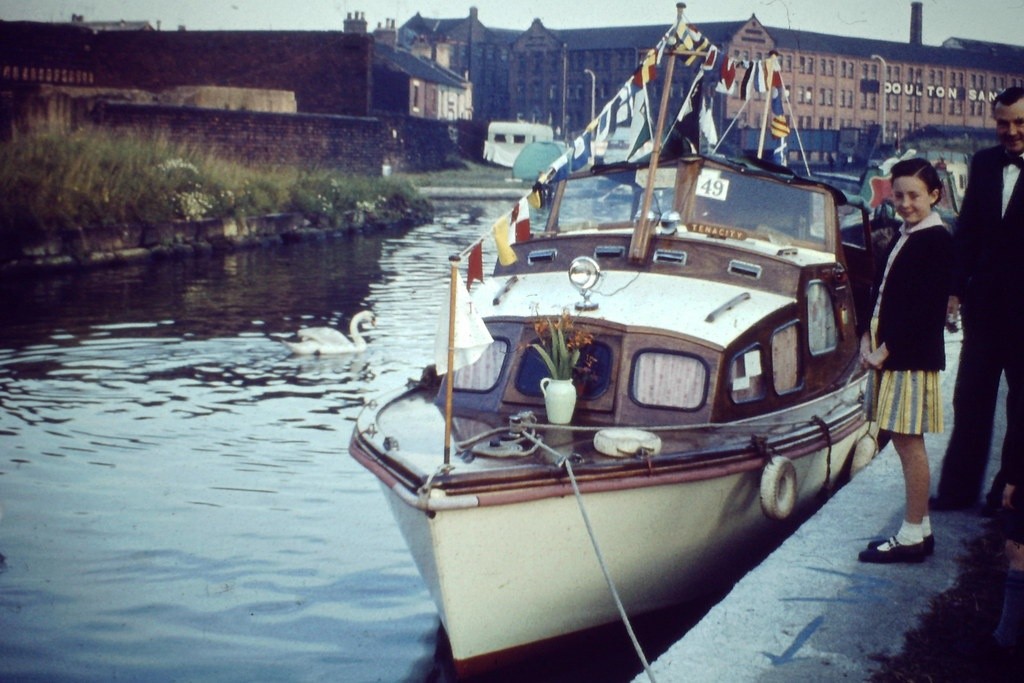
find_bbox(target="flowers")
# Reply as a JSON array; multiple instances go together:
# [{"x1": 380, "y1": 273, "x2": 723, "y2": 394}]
[{"x1": 519, "y1": 300, "x2": 598, "y2": 378}]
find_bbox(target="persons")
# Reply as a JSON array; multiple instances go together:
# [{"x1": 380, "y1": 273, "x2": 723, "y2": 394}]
[
  {"x1": 857, "y1": 157, "x2": 961, "y2": 562},
  {"x1": 927, "y1": 87, "x2": 1023, "y2": 683}
]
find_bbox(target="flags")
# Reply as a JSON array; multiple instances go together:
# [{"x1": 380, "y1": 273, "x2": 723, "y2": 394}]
[{"x1": 430, "y1": 12, "x2": 791, "y2": 379}]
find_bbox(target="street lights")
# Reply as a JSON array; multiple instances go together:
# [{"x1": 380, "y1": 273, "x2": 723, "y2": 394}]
[
  {"x1": 870, "y1": 53, "x2": 887, "y2": 147},
  {"x1": 583, "y1": 68, "x2": 595, "y2": 124}
]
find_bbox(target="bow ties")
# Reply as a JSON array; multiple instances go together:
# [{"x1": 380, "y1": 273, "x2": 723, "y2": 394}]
[{"x1": 998, "y1": 155, "x2": 1024, "y2": 170}]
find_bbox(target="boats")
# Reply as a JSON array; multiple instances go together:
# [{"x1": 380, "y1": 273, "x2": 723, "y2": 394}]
[
  {"x1": 348, "y1": 3, "x2": 962, "y2": 681},
  {"x1": 810, "y1": 147, "x2": 968, "y2": 223}
]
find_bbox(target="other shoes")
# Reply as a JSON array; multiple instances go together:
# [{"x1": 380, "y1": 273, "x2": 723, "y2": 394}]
[
  {"x1": 968, "y1": 636, "x2": 1020, "y2": 669},
  {"x1": 930, "y1": 490, "x2": 981, "y2": 511}
]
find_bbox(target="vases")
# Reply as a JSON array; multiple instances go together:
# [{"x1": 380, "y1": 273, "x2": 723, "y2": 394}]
[{"x1": 542, "y1": 380, "x2": 578, "y2": 423}]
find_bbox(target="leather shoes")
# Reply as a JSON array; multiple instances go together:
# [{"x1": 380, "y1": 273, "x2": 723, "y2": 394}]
[{"x1": 858, "y1": 534, "x2": 935, "y2": 562}]
